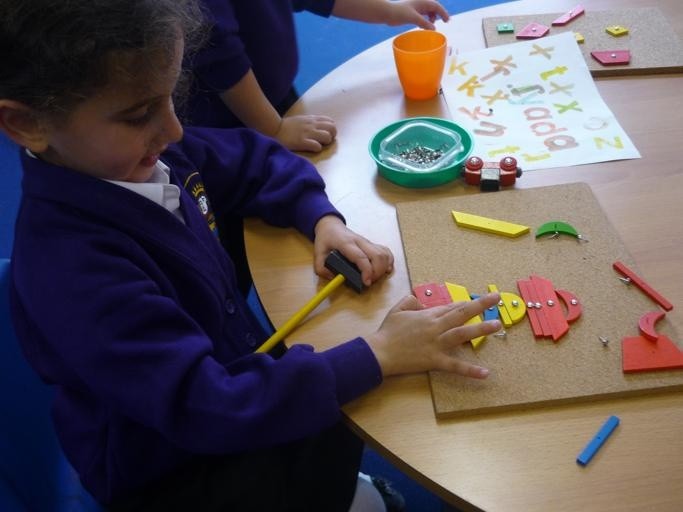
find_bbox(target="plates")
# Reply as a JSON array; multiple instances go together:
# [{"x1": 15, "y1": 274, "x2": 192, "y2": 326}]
[{"x1": 370, "y1": 117, "x2": 472, "y2": 188}]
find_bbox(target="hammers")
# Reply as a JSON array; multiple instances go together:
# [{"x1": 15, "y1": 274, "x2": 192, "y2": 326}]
[{"x1": 254, "y1": 249, "x2": 364, "y2": 355}]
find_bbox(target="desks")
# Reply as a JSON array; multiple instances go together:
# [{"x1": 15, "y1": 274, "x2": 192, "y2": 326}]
[{"x1": 241, "y1": 1, "x2": 683, "y2": 511}]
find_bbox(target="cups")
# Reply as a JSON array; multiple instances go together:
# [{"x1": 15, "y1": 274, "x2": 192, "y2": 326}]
[{"x1": 392, "y1": 28, "x2": 448, "y2": 101}]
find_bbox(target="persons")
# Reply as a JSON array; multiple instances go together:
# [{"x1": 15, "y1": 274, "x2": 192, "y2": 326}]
[
  {"x1": 173, "y1": 0, "x2": 451, "y2": 304},
  {"x1": 0, "y1": 0, "x2": 501, "y2": 510}
]
